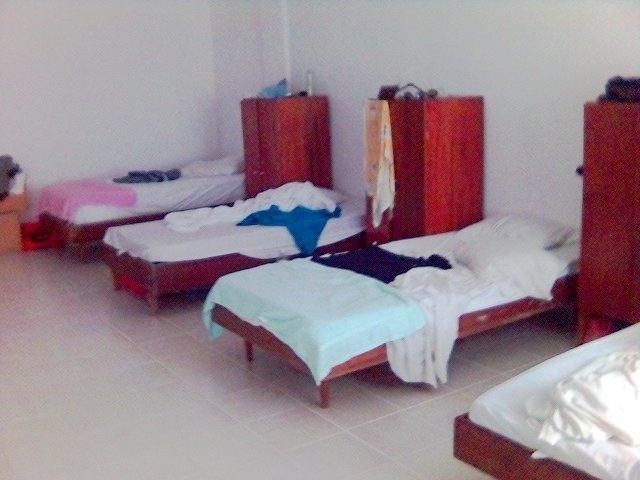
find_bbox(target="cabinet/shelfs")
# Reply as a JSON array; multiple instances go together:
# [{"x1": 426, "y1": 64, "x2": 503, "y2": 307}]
[
  {"x1": 364, "y1": 94, "x2": 486, "y2": 249},
  {"x1": 240, "y1": 94, "x2": 333, "y2": 200},
  {"x1": 576, "y1": 100, "x2": 640, "y2": 347}
]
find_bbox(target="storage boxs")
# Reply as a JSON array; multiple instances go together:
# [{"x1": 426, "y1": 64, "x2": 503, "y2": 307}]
[
  {"x1": 0, "y1": 212, "x2": 24, "y2": 255},
  {"x1": 0, "y1": 172, "x2": 28, "y2": 214},
  {"x1": 21, "y1": 222, "x2": 64, "y2": 250}
]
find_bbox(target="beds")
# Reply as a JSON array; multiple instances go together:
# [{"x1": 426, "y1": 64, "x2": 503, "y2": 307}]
[
  {"x1": 33, "y1": 172, "x2": 247, "y2": 263},
  {"x1": 201, "y1": 232, "x2": 581, "y2": 410},
  {"x1": 454, "y1": 320, "x2": 640, "y2": 480},
  {"x1": 96, "y1": 198, "x2": 366, "y2": 315}
]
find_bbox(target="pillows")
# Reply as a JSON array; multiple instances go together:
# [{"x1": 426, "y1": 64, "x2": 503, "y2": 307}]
[
  {"x1": 319, "y1": 188, "x2": 346, "y2": 204},
  {"x1": 181, "y1": 151, "x2": 245, "y2": 177},
  {"x1": 454, "y1": 211, "x2": 574, "y2": 250}
]
